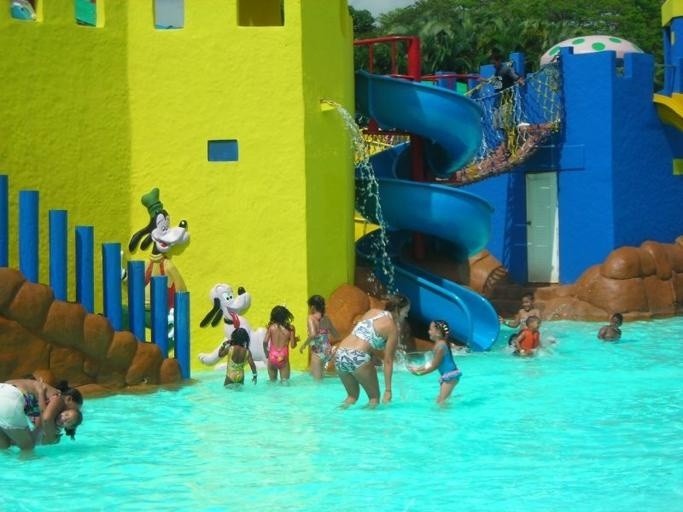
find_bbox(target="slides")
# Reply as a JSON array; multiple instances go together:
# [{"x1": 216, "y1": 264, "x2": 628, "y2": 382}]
[{"x1": 355, "y1": 69, "x2": 500, "y2": 352}]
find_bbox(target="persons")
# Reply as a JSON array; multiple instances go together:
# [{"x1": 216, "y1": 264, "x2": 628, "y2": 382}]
[
  {"x1": 409, "y1": 320, "x2": 462, "y2": 402},
  {"x1": 475, "y1": 47, "x2": 525, "y2": 163},
  {"x1": 335, "y1": 295, "x2": 410, "y2": 405},
  {"x1": 218, "y1": 328, "x2": 257, "y2": 385},
  {"x1": 499, "y1": 293, "x2": 541, "y2": 354},
  {"x1": 263, "y1": 305, "x2": 299, "y2": 381},
  {"x1": 598, "y1": 313, "x2": 623, "y2": 342},
  {"x1": 300, "y1": 295, "x2": 337, "y2": 381},
  {"x1": 0, "y1": 379, "x2": 82, "y2": 451}
]
